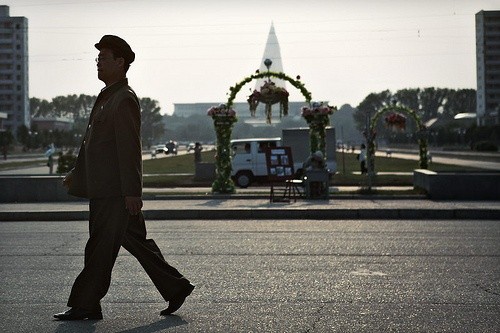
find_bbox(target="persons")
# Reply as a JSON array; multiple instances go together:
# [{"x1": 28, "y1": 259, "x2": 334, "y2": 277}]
[
  {"x1": 47, "y1": 151, "x2": 74, "y2": 175},
  {"x1": 358, "y1": 145, "x2": 369, "y2": 173},
  {"x1": 53, "y1": 35, "x2": 195, "y2": 320},
  {"x1": 193, "y1": 142, "x2": 202, "y2": 163},
  {"x1": 302, "y1": 150, "x2": 325, "y2": 198}
]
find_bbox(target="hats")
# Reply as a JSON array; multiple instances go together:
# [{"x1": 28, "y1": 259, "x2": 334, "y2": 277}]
[{"x1": 95, "y1": 35, "x2": 135, "y2": 63}]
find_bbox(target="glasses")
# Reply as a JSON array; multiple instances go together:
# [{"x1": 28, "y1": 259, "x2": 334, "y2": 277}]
[{"x1": 96, "y1": 57, "x2": 119, "y2": 62}]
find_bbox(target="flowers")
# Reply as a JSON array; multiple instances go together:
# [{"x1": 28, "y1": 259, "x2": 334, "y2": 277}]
[
  {"x1": 208, "y1": 104, "x2": 235, "y2": 116},
  {"x1": 302, "y1": 106, "x2": 330, "y2": 116},
  {"x1": 247, "y1": 81, "x2": 289, "y2": 124}
]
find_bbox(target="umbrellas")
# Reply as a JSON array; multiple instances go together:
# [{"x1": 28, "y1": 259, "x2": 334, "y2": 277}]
[{"x1": 44, "y1": 148, "x2": 55, "y2": 156}]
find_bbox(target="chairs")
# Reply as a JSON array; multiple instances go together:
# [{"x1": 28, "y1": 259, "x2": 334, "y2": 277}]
[{"x1": 284, "y1": 169, "x2": 305, "y2": 202}]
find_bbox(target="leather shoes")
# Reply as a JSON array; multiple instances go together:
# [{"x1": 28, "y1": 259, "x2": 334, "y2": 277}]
[
  {"x1": 54, "y1": 307, "x2": 103, "y2": 321},
  {"x1": 160, "y1": 284, "x2": 195, "y2": 315}
]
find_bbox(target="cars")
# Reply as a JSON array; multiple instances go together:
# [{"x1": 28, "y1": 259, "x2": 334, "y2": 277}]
[
  {"x1": 155, "y1": 144, "x2": 166, "y2": 153},
  {"x1": 164, "y1": 143, "x2": 177, "y2": 155},
  {"x1": 187, "y1": 143, "x2": 195, "y2": 152}
]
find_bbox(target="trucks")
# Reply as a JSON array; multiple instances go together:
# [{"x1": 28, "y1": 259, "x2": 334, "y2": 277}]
[{"x1": 228, "y1": 127, "x2": 338, "y2": 188}]
